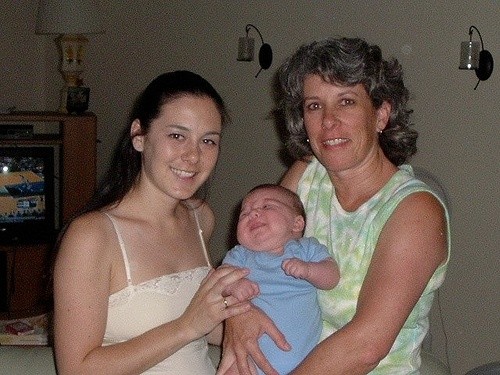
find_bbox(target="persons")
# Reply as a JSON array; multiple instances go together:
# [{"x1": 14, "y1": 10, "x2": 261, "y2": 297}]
[
  {"x1": 212, "y1": 37, "x2": 452, "y2": 375},
  {"x1": 53, "y1": 69, "x2": 251, "y2": 375},
  {"x1": 215, "y1": 183, "x2": 340, "y2": 375}
]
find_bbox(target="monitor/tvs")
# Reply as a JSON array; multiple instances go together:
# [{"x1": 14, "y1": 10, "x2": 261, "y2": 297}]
[{"x1": 0, "y1": 143, "x2": 62, "y2": 239}]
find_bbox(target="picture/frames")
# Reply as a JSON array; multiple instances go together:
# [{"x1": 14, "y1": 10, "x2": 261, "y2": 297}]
[{"x1": 66, "y1": 86, "x2": 90, "y2": 112}]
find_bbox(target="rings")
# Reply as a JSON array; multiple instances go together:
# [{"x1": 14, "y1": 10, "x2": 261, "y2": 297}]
[{"x1": 223, "y1": 298, "x2": 229, "y2": 309}]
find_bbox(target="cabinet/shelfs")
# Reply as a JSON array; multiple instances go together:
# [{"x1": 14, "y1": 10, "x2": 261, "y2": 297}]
[{"x1": 0, "y1": 112, "x2": 97, "y2": 317}]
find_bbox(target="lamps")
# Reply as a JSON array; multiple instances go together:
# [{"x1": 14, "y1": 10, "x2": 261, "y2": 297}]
[
  {"x1": 237, "y1": 24, "x2": 273, "y2": 79},
  {"x1": 459, "y1": 25, "x2": 494, "y2": 90},
  {"x1": 36, "y1": 0, "x2": 108, "y2": 115}
]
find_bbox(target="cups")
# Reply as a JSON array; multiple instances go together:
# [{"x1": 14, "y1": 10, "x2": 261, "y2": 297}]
[{"x1": 66, "y1": 85, "x2": 90, "y2": 115}]
